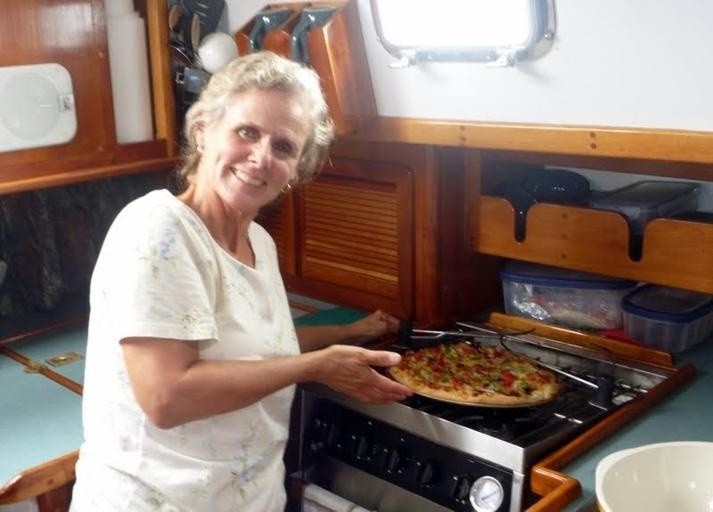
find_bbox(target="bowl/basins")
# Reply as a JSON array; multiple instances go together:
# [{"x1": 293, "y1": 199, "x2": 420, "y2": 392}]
[{"x1": 593, "y1": 440, "x2": 713, "y2": 512}]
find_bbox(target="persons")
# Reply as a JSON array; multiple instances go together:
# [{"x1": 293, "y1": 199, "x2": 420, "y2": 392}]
[{"x1": 63, "y1": 48, "x2": 415, "y2": 511}]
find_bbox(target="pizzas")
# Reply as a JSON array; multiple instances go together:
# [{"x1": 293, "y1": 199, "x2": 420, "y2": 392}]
[{"x1": 390, "y1": 342, "x2": 561, "y2": 406}]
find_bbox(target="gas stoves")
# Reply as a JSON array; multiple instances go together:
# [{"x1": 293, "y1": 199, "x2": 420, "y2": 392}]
[{"x1": 294, "y1": 315, "x2": 657, "y2": 512}]
